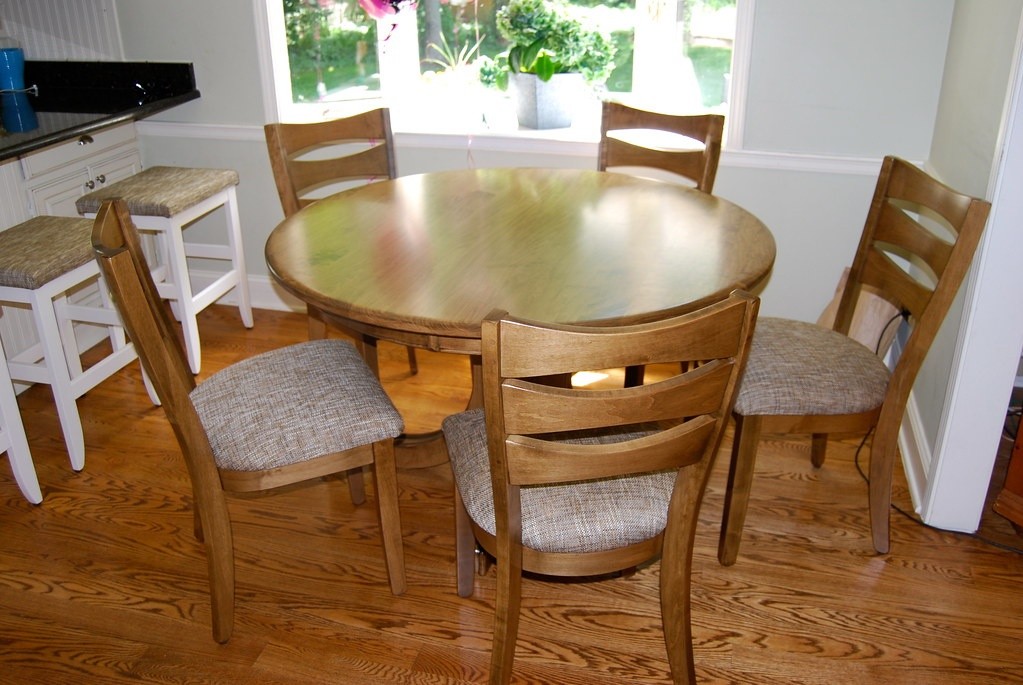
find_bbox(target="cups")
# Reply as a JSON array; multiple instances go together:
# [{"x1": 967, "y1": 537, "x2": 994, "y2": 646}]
[
  {"x1": 1, "y1": 49, "x2": 24, "y2": 93},
  {"x1": 3, "y1": 94, "x2": 38, "y2": 131}
]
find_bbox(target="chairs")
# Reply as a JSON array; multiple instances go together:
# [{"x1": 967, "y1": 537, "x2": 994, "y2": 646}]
[
  {"x1": 265, "y1": 98, "x2": 991, "y2": 567},
  {"x1": 88, "y1": 195, "x2": 407, "y2": 643},
  {"x1": 441, "y1": 289, "x2": 761, "y2": 685}
]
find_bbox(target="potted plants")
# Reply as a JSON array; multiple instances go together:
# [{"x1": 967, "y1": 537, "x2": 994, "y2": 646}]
[{"x1": 421, "y1": 1, "x2": 617, "y2": 131}]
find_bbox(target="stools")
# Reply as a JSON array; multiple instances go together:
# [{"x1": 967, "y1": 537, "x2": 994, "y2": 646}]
[
  {"x1": 75, "y1": 165, "x2": 253, "y2": 375},
  {"x1": 0, "y1": 215, "x2": 161, "y2": 505}
]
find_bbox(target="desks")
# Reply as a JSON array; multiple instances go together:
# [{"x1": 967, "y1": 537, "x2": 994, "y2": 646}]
[{"x1": 265, "y1": 166, "x2": 776, "y2": 577}]
